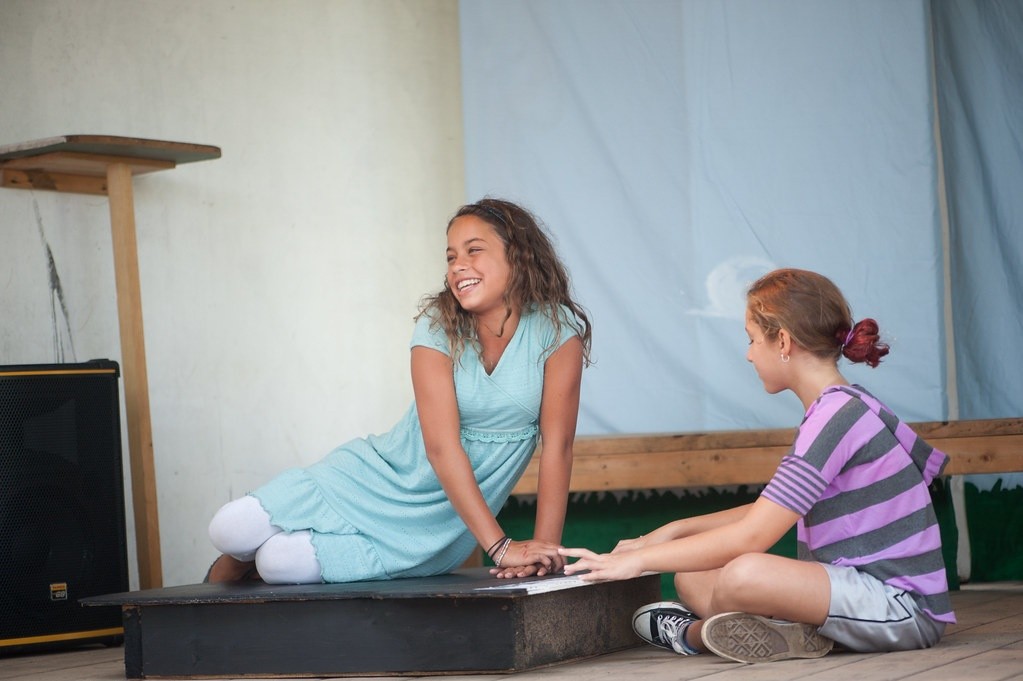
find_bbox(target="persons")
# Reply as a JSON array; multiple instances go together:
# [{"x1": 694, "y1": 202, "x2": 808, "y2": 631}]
[
  {"x1": 200, "y1": 199, "x2": 597, "y2": 586},
  {"x1": 558, "y1": 267, "x2": 957, "y2": 665}
]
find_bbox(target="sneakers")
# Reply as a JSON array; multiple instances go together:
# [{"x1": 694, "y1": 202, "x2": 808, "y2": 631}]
[
  {"x1": 701, "y1": 611, "x2": 834, "y2": 663},
  {"x1": 632, "y1": 601, "x2": 699, "y2": 655}
]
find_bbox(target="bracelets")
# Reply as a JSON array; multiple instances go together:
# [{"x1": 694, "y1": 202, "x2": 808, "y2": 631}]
[{"x1": 487, "y1": 535, "x2": 513, "y2": 567}]
